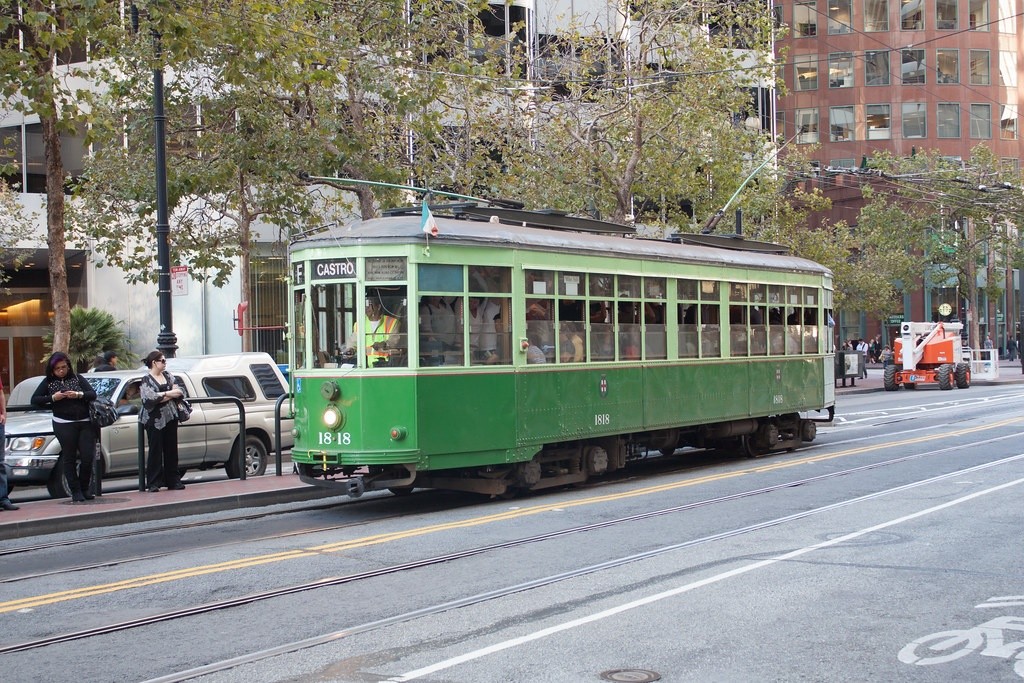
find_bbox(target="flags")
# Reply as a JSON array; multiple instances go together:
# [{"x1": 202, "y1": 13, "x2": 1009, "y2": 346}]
[{"x1": 420, "y1": 200, "x2": 439, "y2": 237}]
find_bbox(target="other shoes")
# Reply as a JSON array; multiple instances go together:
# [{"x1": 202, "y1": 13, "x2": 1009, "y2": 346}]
[
  {"x1": 83, "y1": 493, "x2": 95, "y2": 499},
  {"x1": 150, "y1": 486, "x2": 159, "y2": 492},
  {"x1": 72, "y1": 494, "x2": 85, "y2": 501},
  {"x1": 865, "y1": 375, "x2": 867, "y2": 378},
  {"x1": 0, "y1": 499, "x2": 19, "y2": 511},
  {"x1": 858, "y1": 378, "x2": 863, "y2": 379},
  {"x1": 168, "y1": 485, "x2": 185, "y2": 489}
]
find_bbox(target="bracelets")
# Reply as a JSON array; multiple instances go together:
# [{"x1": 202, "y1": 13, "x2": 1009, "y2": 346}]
[
  {"x1": 165, "y1": 390, "x2": 166, "y2": 396},
  {"x1": 76, "y1": 391, "x2": 78, "y2": 398},
  {"x1": 52, "y1": 395, "x2": 55, "y2": 402}
]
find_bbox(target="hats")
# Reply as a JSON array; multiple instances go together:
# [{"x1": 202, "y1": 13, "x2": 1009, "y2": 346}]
[
  {"x1": 104, "y1": 351, "x2": 118, "y2": 359},
  {"x1": 98, "y1": 352, "x2": 105, "y2": 357}
]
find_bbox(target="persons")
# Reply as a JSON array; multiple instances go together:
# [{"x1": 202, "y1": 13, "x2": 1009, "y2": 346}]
[
  {"x1": 138, "y1": 351, "x2": 185, "y2": 492},
  {"x1": 87, "y1": 351, "x2": 119, "y2": 373},
  {"x1": 879, "y1": 345, "x2": 894, "y2": 369},
  {"x1": 30, "y1": 352, "x2": 97, "y2": 502},
  {"x1": 347, "y1": 288, "x2": 401, "y2": 368},
  {"x1": 0, "y1": 376, "x2": 20, "y2": 511},
  {"x1": 984, "y1": 335, "x2": 993, "y2": 360},
  {"x1": 841, "y1": 337, "x2": 868, "y2": 379},
  {"x1": 119, "y1": 383, "x2": 141, "y2": 412},
  {"x1": 868, "y1": 336, "x2": 882, "y2": 364},
  {"x1": 1006, "y1": 336, "x2": 1018, "y2": 361},
  {"x1": 419, "y1": 265, "x2": 835, "y2": 363}
]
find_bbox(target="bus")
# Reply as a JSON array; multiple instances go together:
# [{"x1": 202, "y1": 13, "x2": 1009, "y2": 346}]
[{"x1": 287, "y1": 202, "x2": 835, "y2": 497}]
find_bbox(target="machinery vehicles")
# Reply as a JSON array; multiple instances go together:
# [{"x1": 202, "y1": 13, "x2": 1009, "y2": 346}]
[{"x1": 883, "y1": 321, "x2": 971, "y2": 389}]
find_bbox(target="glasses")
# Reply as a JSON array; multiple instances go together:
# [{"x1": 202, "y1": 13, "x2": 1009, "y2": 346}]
[
  {"x1": 156, "y1": 359, "x2": 165, "y2": 364},
  {"x1": 53, "y1": 365, "x2": 68, "y2": 371}
]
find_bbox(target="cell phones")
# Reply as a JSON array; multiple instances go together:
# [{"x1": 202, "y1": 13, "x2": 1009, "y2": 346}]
[{"x1": 60, "y1": 389, "x2": 71, "y2": 394}]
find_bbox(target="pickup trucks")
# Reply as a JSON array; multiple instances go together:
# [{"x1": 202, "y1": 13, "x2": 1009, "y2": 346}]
[{"x1": 2, "y1": 353, "x2": 295, "y2": 498}]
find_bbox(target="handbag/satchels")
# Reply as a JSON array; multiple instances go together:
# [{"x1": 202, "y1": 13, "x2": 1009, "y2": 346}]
[
  {"x1": 163, "y1": 372, "x2": 192, "y2": 423},
  {"x1": 74, "y1": 372, "x2": 119, "y2": 426}
]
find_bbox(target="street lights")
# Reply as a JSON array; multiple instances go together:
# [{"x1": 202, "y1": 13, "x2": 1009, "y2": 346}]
[{"x1": 144, "y1": 0, "x2": 179, "y2": 358}]
